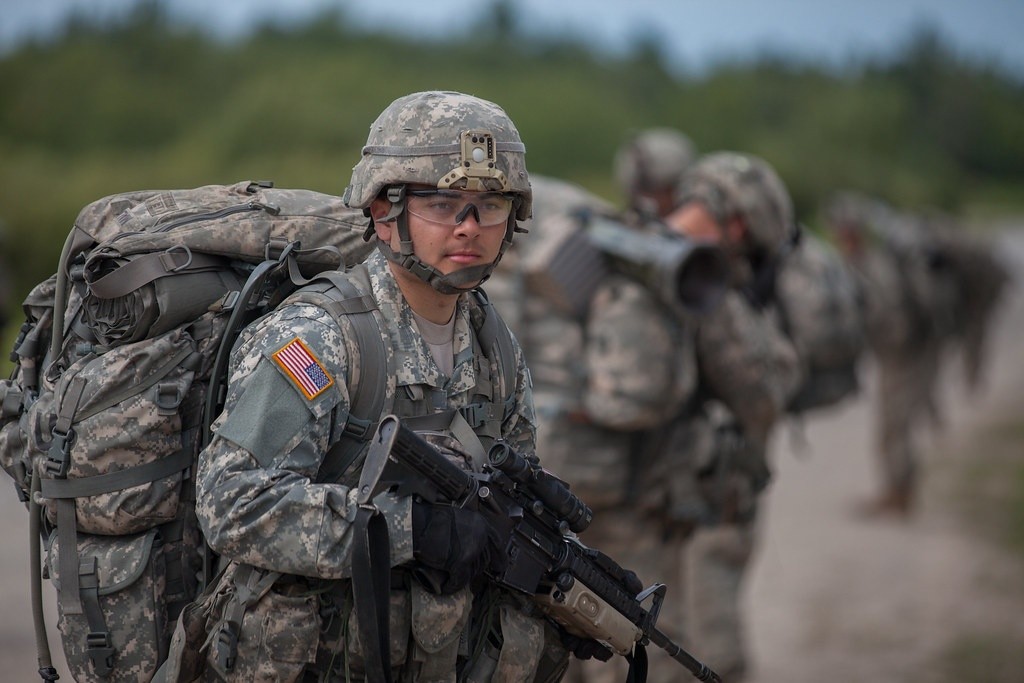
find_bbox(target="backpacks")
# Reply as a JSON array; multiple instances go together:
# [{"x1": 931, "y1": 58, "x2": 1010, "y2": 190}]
[
  {"x1": 0, "y1": 179, "x2": 518, "y2": 683},
  {"x1": 484, "y1": 173, "x2": 698, "y2": 507},
  {"x1": 774, "y1": 224, "x2": 861, "y2": 410}
]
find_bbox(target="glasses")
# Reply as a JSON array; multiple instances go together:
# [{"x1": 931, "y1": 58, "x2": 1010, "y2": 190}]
[{"x1": 377, "y1": 189, "x2": 516, "y2": 227}]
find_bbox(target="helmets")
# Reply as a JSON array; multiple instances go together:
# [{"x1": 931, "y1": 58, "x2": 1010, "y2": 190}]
[
  {"x1": 671, "y1": 148, "x2": 796, "y2": 250},
  {"x1": 615, "y1": 126, "x2": 697, "y2": 199},
  {"x1": 343, "y1": 90, "x2": 533, "y2": 221}
]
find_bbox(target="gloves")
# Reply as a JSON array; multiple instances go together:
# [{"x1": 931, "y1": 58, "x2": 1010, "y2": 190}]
[
  {"x1": 558, "y1": 569, "x2": 643, "y2": 663},
  {"x1": 418, "y1": 502, "x2": 515, "y2": 596}
]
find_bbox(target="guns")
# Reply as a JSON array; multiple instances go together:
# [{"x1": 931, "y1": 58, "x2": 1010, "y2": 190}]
[{"x1": 354, "y1": 415, "x2": 727, "y2": 683}]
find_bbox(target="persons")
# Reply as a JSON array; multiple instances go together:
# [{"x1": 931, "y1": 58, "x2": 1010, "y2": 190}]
[
  {"x1": 575, "y1": 127, "x2": 943, "y2": 683},
  {"x1": 193, "y1": 91, "x2": 642, "y2": 683}
]
